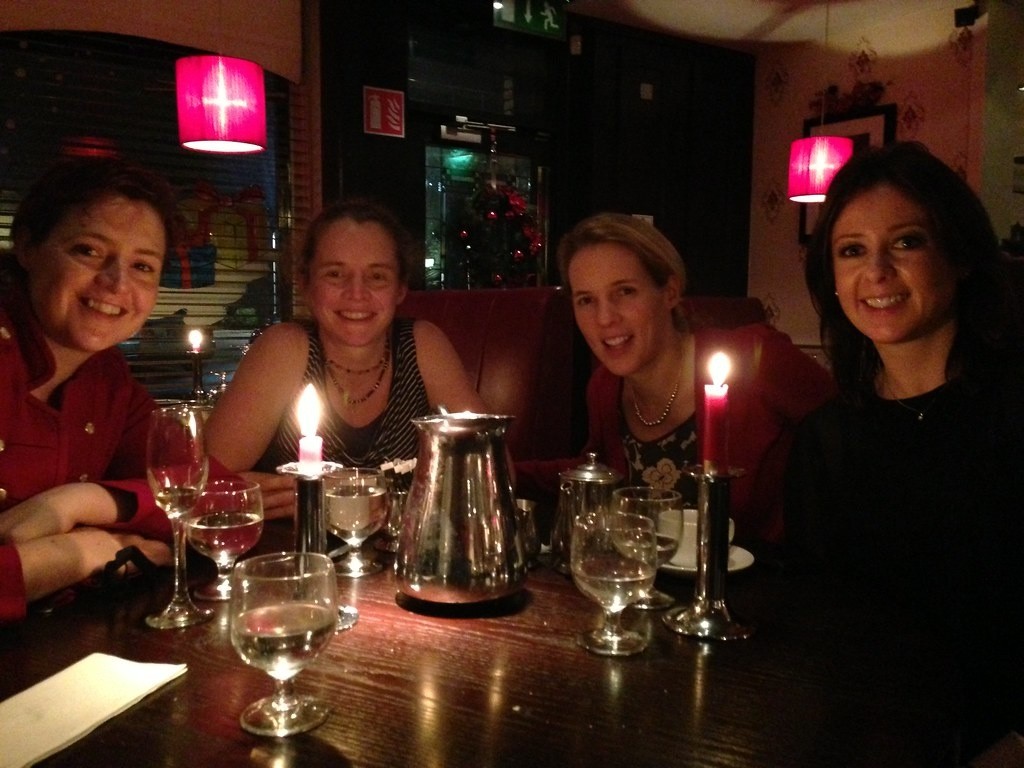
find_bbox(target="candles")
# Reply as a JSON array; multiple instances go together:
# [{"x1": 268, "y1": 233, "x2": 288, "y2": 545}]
[
  {"x1": 704, "y1": 384, "x2": 729, "y2": 473},
  {"x1": 299, "y1": 436, "x2": 322, "y2": 472}
]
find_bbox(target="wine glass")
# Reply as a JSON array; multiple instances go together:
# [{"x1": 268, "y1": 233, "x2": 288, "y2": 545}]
[
  {"x1": 322, "y1": 468, "x2": 389, "y2": 579},
  {"x1": 611, "y1": 486, "x2": 684, "y2": 611},
  {"x1": 186, "y1": 480, "x2": 265, "y2": 601},
  {"x1": 229, "y1": 550, "x2": 340, "y2": 735},
  {"x1": 571, "y1": 512, "x2": 658, "y2": 657},
  {"x1": 145, "y1": 407, "x2": 215, "y2": 630}
]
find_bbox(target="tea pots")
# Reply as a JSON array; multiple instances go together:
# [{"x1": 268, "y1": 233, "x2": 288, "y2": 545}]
[{"x1": 550, "y1": 451, "x2": 625, "y2": 574}]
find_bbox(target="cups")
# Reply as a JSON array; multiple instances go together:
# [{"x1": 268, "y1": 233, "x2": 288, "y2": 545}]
[
  {"x1": 516, "y1": 497, "x2": 540, "y2": 568},
  {"x1": 658, "y1": 508, "x2": 734, "y2": 569}
]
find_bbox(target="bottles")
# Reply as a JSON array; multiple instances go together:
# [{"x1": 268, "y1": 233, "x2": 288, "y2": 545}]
[{"x1": 394, "y1": 411, "x2": 526, "y2": 607}]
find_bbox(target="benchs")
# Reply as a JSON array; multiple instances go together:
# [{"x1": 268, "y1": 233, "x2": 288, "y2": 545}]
[{"x1": 391, "y1": 288, "x2": 760, "y2": 526}]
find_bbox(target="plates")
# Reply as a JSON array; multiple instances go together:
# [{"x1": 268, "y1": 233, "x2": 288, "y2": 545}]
[{"x1": 661, "y1": 545, "x2": 755, "y2": 573}]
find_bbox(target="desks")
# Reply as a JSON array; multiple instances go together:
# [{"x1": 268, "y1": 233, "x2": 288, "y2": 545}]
[{"x1": 0, "y1": 520, "x2": 848, "y2": 768}]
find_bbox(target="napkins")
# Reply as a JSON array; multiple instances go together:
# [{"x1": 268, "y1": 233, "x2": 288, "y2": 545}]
[{"x1": 0, "y1": 652, "x2": 189, "y2": 768}]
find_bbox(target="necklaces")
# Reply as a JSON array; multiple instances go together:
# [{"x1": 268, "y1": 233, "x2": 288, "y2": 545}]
[
  {"x1": 887, "y1": 386, "x2": 926, "y2": 420},
  {"x1": 325, "y1": 329, "x2": 391, "y2": 404},
  {"x1": 631, "y1": 332, "x2": 685, "y2": 426}
]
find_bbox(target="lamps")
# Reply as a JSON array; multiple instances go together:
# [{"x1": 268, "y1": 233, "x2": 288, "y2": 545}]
[
  {"x1": 786, "y1": 0, "x2": 852, "y2": 203},
  {"x1": 175, "y1": 0, "x2": 267, "y2": 154}
]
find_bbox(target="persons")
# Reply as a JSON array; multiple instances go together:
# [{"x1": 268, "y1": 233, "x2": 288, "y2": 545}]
[
  {"x1": 782, "y1": 141, "x2": 1024, "y2": 768},
  {"x1": 202, "y1": 197, "x2": 486, "y2": 516},
  {"x1": 514, "y1": 212, "x2": 835, "y2": 542},
  {"x1": 0, "y1": 156, "x2": 244, "y2": 620}
]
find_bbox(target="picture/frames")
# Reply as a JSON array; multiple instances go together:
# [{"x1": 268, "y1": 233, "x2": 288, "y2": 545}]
[{"x1": 799, "y1": 103, "x2": 899, "y2": 242}]
